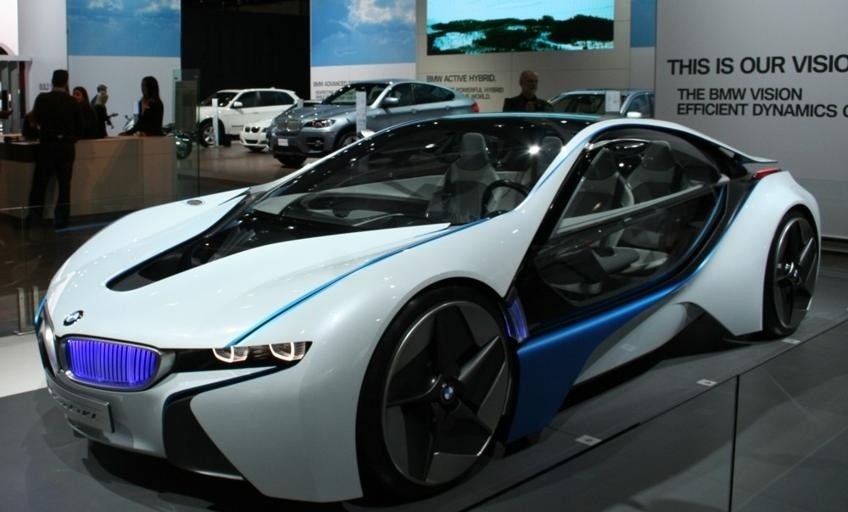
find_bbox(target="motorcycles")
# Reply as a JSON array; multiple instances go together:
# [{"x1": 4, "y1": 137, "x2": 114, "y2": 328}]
[{"x1": 119, "y1": 114, "x2": 193, "y2": 159}]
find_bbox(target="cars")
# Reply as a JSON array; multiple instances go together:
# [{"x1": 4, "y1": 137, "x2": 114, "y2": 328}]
[
  {"x1": 239, "y1": 100, "x2": 357, "y2": 152},
  {"x1": 265, "y1": 78, "x2": 481, "y2": 168},
  {"x1": 547, "y1": 87, "x2": 655, "y2": 117}
]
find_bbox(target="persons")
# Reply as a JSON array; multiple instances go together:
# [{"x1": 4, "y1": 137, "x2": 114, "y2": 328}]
[
  {"x1": 94, "y1": 95, "x2": 118, "y2": 130},
  {"x1": 92, "y1": 84, "x2": 119, "y2": 139},
  {"x1": 9, "y1": 68, "x2": 78, "y2": 232},
  {"x1": 0, "y1": 110, "x2": 13, "y2": 120},
  {"x1": 72, "y1": 85, "x2": 108, "y2": 140},
  {"x1": 500, "y1": 69, "x2": 556, "y2": 113},
  {"x1": 119, "y1": 76, "x2": 167, "y2": 136}
]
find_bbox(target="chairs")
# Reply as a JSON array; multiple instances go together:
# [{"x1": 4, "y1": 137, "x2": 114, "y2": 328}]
[
  {"x1": 426, "y1": 129, "x2": 501, "y2": 222},
  {"x1": 515, "y1": 135, "x2": 687, "y2": 278}
]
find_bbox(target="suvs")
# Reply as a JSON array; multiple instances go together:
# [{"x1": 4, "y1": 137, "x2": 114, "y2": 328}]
[{"x1": 195, "y1": 88, "x2": 301, "y2": 149}]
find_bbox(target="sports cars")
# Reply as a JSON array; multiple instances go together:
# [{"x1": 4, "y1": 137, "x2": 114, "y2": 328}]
[{"x1": 29, "y1": 112, "x2": 824, "y2": 508}]
